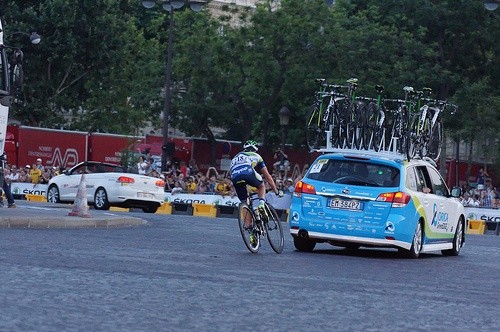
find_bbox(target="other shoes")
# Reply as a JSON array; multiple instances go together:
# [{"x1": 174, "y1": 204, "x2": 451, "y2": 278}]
[{"x1": 9, "y1": 203, "x2": 15, "y2": 207}]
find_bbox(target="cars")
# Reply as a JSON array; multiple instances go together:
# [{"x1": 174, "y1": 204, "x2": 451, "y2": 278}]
[{"x1": 287, "y1": 152, "x2": 466, "y2": 256}]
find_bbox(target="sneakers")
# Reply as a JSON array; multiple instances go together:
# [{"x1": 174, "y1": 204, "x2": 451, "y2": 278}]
[
  {"x1": 249, "y1": 235, "x2": 261, "y2": 247},
  {"x1": 258, "y1": 204, "x2": 269, "y2": 223}
]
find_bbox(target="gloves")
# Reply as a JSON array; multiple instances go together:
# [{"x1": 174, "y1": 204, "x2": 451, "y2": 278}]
[{"x1": 276, "y1": 190, "x2": 283, "y2": 198}]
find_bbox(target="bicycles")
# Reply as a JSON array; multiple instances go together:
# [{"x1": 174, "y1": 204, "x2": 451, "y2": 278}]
[
  {"x1": 236, "y1": 188, "x2": 285, "y2": 254},
  {"x1": 304, "y1": 78, "x2": 456, "y2": 162}
]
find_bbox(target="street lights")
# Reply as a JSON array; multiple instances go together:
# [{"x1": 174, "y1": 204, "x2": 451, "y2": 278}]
[{"x1": 142, "y1": 0, "x2": 207, "y2": 164}]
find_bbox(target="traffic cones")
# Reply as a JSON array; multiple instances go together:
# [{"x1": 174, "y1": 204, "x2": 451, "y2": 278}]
[{"x1": 69, "y1": 173, "x2": 93, "y2": 218}]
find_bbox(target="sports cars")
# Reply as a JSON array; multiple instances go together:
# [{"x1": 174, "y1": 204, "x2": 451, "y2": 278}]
[{"x1": 47, "y1": 162, "x2": 166, "y2": 214}]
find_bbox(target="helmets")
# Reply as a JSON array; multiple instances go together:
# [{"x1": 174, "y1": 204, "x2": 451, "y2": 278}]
[{"x1": 243, "y1": 140, "x2": 258, "y2": 151}]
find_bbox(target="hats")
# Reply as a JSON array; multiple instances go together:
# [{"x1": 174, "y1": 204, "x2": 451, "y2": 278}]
[
  {"x1": 26, "y1": 165, "x2": 30, "y2": 168},
  {"x1": 37, "y1": 158, "x2": 42, "y2": 162},
  {"x1": 288, "y1": 179, "x2": 292, "y2": 182}
]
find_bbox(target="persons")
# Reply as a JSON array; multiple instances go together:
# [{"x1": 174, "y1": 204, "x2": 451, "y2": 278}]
[
  {"x1": 0, "y1": 149, "x2": 304, "y2": 208},
  {"x1": 229, "y1": 140, "x2": 284, "y2": 247},
  {"x1": 356, "y1": 164, "x2": 500, "y2": 209}
]
